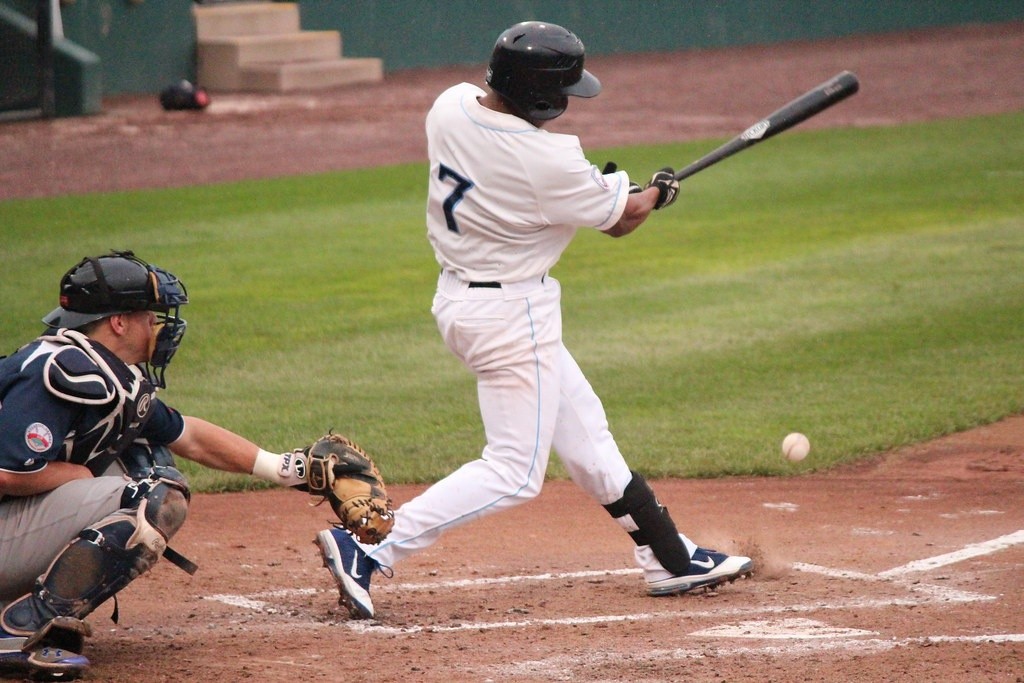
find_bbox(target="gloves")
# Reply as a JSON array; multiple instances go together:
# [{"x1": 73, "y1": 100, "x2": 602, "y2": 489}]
[
  {"x1": 643, "y1": 166, "x2": 681, "y2": 209},
  {"x1": 601, "y1": 161, "x2": 642, "y2": 195}
]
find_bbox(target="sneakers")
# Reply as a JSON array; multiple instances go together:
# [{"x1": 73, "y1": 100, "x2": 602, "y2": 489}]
[
  {"x1": 634, "y1": 531, "x2": 754, "y2": 597},
  {"x1": 312, "y1": 525, "x2": 393, "y2": 621},
  {"x1": 0, "y1": 629, "x2": 94, "y2": 678}
]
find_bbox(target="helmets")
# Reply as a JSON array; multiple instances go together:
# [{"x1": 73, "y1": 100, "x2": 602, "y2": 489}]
[
  {"x1": 41, "y1": 250, "x2": 163, "y2": 330},
  {"x1": 158, "y1": 78, "x2": 212, "y2": 110},
  {"x1": 485, "y1": 19, "x2": 603, "y2": 121}
]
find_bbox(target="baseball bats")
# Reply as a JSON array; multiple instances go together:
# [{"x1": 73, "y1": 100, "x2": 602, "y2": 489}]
[{"x1": 644, "y1": 68, "x2": 860, "y2": 183}]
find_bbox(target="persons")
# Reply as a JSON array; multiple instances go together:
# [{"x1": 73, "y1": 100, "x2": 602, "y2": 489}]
[
  {"x1": 315, "y1": 21, "x2": 752, "y2": 619},
  {"x1": 1, "y1": 253, "x2": 393, "y2": 683}
]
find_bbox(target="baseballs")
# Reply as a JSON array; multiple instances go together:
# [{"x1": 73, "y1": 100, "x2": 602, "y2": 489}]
[{"x1": 780, "y1": 430, "x2": 812, "y2": 461}]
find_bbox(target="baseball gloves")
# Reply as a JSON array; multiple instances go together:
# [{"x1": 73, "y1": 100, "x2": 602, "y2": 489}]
[{"x1": 308, "y1": 428, "x2": 396, "y2": 546}]
